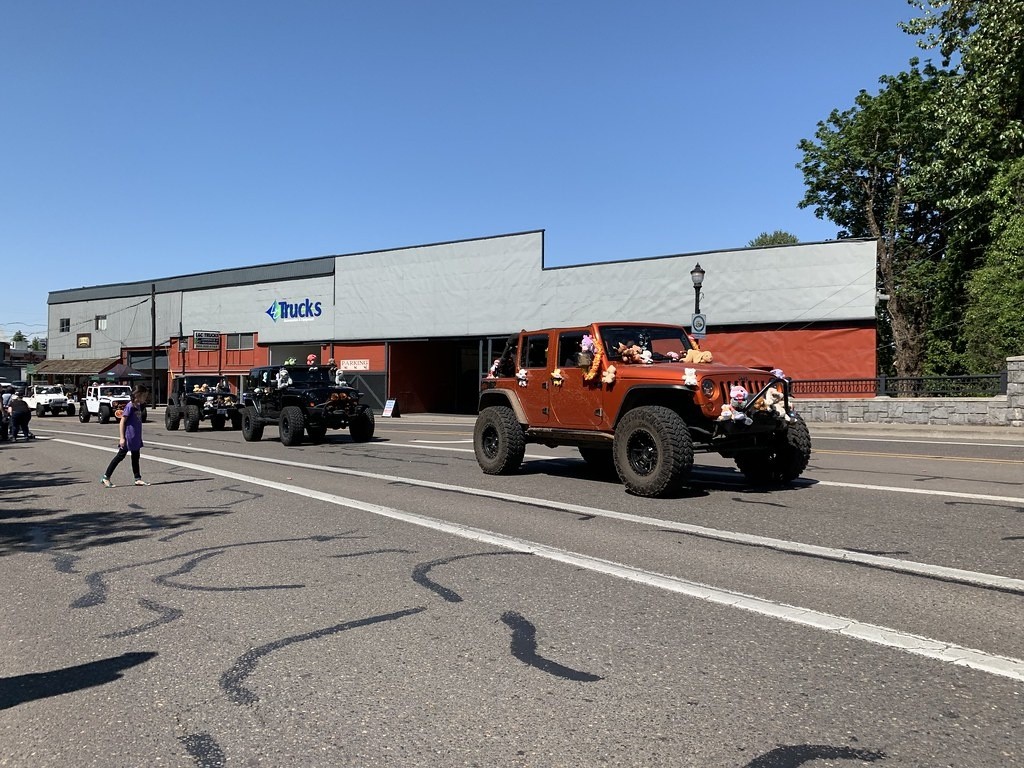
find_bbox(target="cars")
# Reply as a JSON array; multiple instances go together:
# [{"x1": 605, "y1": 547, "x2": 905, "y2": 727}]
[{"x1": 10, "y1": 379, "x2": 27, "y2": 395}]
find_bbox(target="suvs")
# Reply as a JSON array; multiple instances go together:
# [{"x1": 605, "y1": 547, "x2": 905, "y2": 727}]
[
  {"x1": 165, "y1": 376, "x2": 242, "y2": 434},
  {"x1": 241, "y1": 364, "x2": 375, "y2": 446},
  {"x1": 22, "y1": 383, "x2": 75, "y2": 417},
  {"x1": 476, "y1": 317, "x2": 811, "y2": 499},
  {"x1": 77, "y1": 384, "x2": 149, "y2": 425},
  {"x1": 0, "y1": 377, "x2": 11, "y2": 393}
]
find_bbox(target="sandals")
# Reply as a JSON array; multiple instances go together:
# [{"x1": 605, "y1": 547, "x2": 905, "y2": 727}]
[
  {"x1": 100, "y1": 476, "x2": 116, "y2": 488},
  {"x1": 135, "y1": 481, "x2": 151, "y2": 487}
]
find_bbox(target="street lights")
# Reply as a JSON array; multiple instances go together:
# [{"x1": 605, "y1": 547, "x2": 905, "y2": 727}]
[
  {"x1": 180, "y1": 341, "x2": 189, "y2": 375},
  {"x1": 690, "y1": 263, "x2": 708, "y2": 347}
]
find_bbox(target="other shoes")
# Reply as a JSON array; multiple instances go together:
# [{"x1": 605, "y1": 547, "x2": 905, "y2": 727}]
[
  {"x1": 10, "y1": 438, "x2": 16, "y2": 442},
  {"x1": 25, "y1": 437, "x2": 29, "y2": 441}
]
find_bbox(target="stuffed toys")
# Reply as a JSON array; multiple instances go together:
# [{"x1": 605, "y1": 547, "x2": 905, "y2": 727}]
[
  {"x1": 715, "y1": 383, "x2": 798, "y2": 425},
  {"x1": 666, "y1": 350, "x2": 679, "y2": 360},
  {"x1": 770, "y1": 369, "x2": 789, "y2": 390},
  {"x1": 338, "y1": 392, "x2": 350, "y2": 411},
  {"x1": 579, "y1": 334, "x2": 593, "y2": 353},
  {"x1": 335, "y1": 369, "x2": 346, "y2": 387},
  {"x1": 602, "y1": 365, "x2": 617, "y2": 383},
  {"x1": 487, "y1": 359, "x2": 500, "y2": 379},
  {"x1": 325, "y1": 392, "x2": 339, "y2": 410},
  {"x1": 277, "y1": 369, "x2": 293, "y2": 390},
  {"x1": 612, "y1": 342, "x2": 653, "y2": 364},
  {"x1": 306, "y1": 353, "x2": 317, "y2": 365},
  {"x1": 681, "y1": 367, "x2": 698, "y2": 386},
  {"x1": 284, "y1": 356, "x2": 297, "y2": 365},
  {"x1": 679, "y1": 348, "x2": 714, "y2": 364},
  {"x1": 515, "y1": 368, "x2": 528, "y2": 387},
  {"x1": 326, "y1": 357, "x2": 337, "y2": 366},
  {"x1": 347, "y1": 391, "x2": 358, "y2": 408},
  {"x1": 550, "y1": 368, "x2": 564, "y2": 386},
  {"x1": 193, "y1": 382, "x2": 235, "y2": 408}
]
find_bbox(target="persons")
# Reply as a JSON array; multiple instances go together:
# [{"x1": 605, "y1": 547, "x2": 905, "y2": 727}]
[
  {"x1": 1, "y1": 386, "x2": 16, "y2": 435},
  {"x1": 7, "y1": 394, "x2": 32, "y2": 443},
  {"x1": 99, "y1": 383, "x2": 151, "y2": 488},
  {"x1": 0, "y1": 384, "x2": 10, "y2": 441},
  {"x1": 27, "y1": 381, "x2": 132, "y2": 402}
]
